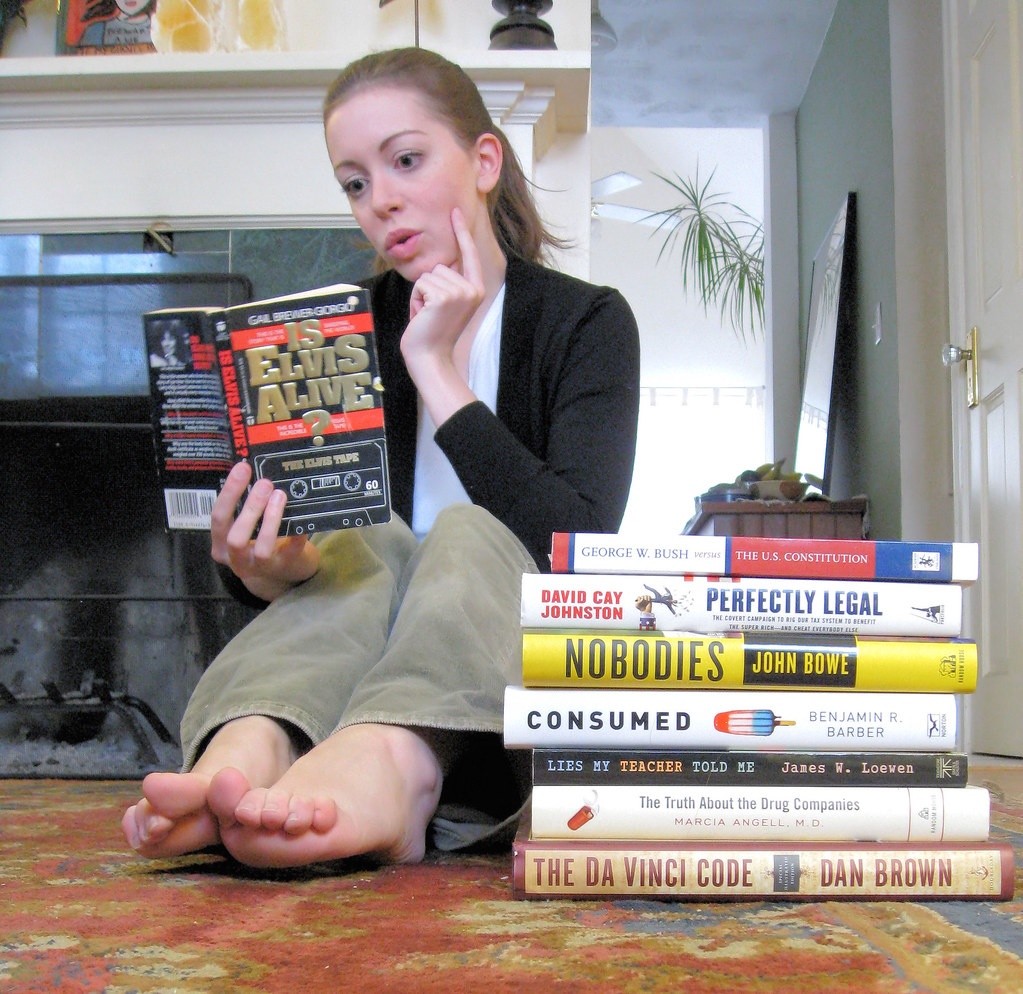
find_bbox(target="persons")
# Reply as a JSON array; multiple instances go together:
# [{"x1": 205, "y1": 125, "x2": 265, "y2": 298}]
[
  {"x1": 150, "y1": 322, "x2": 190, "y2": 367},
  {"x1": 119, "y1": 45, "x2": 641, "y2": 872}
]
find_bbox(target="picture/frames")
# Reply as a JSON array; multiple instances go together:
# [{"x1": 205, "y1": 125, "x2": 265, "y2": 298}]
[
  {"x1": 53, "y1": 0, "x2": 156, "y2": 54},
  {"x1": 793, "y1": 190, "x2": 858, "y2": 498}
]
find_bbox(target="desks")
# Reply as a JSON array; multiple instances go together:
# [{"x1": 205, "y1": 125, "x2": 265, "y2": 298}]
[{"x1": 680, "y1": 495, "x2": 866, "y2": 539}]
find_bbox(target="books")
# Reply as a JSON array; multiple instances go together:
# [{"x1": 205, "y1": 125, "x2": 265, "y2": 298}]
[
  {"x1": 141, "y1": 281, "x2": 393, "y2": 541},
  {"x1": 502, "y1": 531, "x2": 1015, "y2": 903}
]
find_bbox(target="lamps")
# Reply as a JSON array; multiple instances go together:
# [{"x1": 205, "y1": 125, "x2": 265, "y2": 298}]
[{"x1": 591, "y1": 0, "x2": 616, "y2": 58}]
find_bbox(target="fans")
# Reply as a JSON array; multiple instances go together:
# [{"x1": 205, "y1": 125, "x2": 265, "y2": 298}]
[{"x1": 590, "y1": 169, "x2": 684, "y2": 230}]
